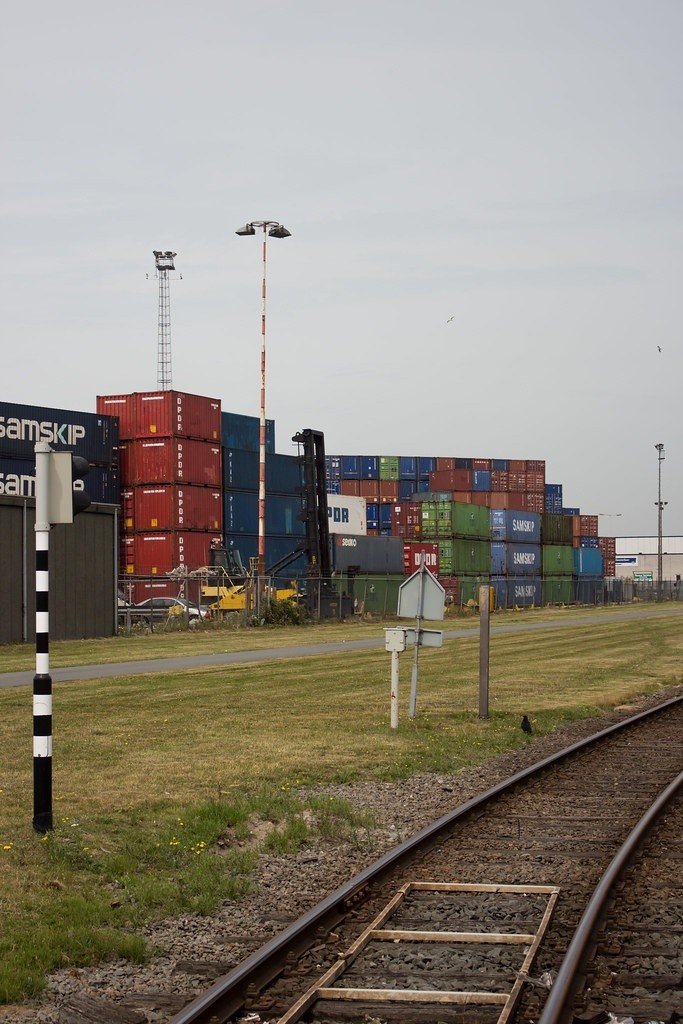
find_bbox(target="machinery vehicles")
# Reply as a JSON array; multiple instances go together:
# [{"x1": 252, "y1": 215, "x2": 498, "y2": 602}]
[{"x1": 166, "y1": 535, "x2": 309, "y2": 622}]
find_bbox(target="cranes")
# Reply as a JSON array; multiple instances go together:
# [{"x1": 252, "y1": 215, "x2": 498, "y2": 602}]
[{"x1": 145, "y1": 248, "x2": 184, "y2": 390}]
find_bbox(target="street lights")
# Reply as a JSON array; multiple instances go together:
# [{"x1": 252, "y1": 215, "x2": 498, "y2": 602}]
[
  {"x1": 654, "y1": 443, "x2": 664, "y2": 601},
  {"x1": 237, "y1": 221, "x2": 292, "y2": 604}
]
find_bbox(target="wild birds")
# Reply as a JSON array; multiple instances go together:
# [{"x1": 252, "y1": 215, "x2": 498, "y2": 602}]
[
  {"x1": 521, "y1": 716, "x2": 532, "y2": 733},
  {"x1": 447, "y1": 317, "x2": 454, "y2": 323},
  {"x1": 657, "y1": 346, "x2": 662, "y2": 352}
]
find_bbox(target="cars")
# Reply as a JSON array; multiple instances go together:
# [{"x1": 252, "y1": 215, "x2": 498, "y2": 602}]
[{"x1": 119, "y1": 596, "x2": 211, "y2": 630}]
